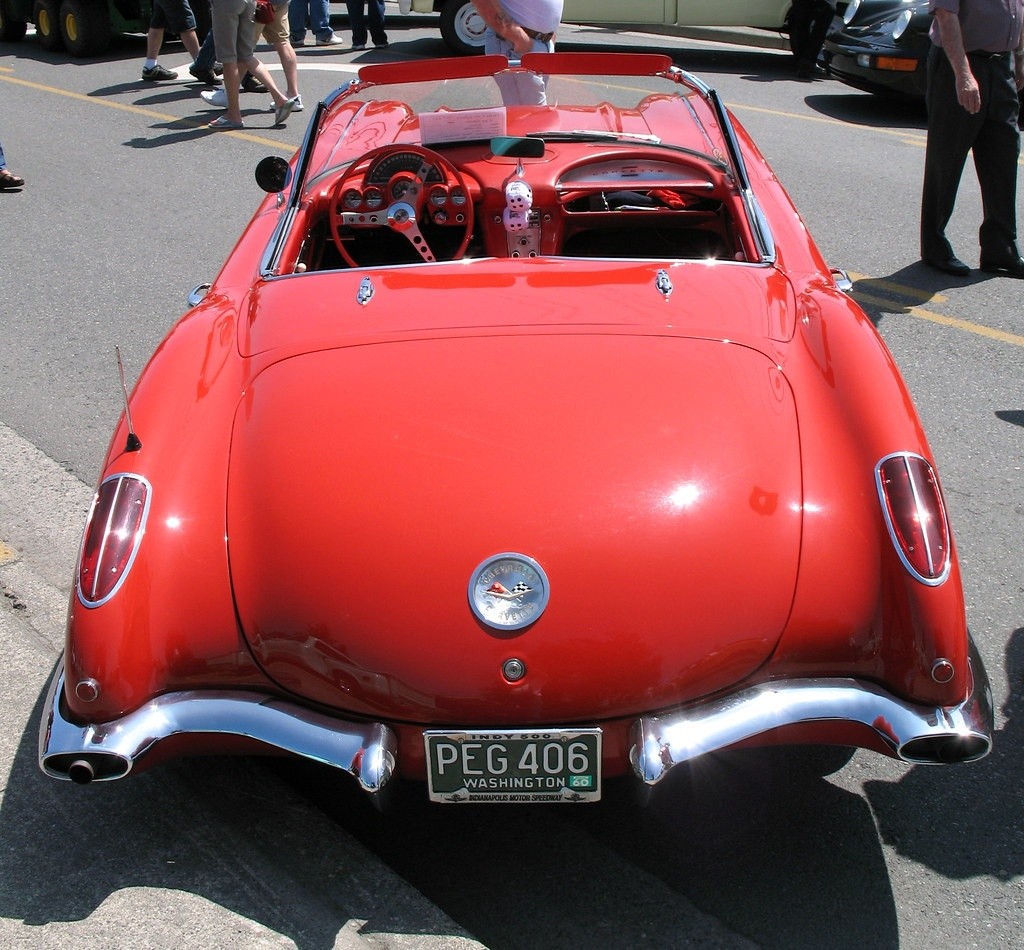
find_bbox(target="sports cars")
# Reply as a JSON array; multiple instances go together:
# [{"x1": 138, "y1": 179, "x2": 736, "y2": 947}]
[{"x1": 38, "y1": 53, "x2": 997, "y2": 807}]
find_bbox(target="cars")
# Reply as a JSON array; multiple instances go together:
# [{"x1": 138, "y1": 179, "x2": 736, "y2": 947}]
[{"x1": 1, "y1": 0, "x2": 1024, "y2": 111}]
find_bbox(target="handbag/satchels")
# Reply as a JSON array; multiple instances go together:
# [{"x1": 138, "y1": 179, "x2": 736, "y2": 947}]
[{"x1": 254, "y1": 0, "x2": 275, "y2": 25}]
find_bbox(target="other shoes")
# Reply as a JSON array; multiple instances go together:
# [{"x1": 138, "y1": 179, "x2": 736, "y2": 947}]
[
  {"x1": 979, "y1": 247, "x2": 1024, "y2": 278},
  {"x1": 922, "y1": 254, "x2": 971, "y2": 276},
  {"x1": 376, "y1": 44, "x2": 389, "y2": 48},
  {"x1": 796, "y1": 62, "x2": 825, "y2": 82},
  {"x1": 351, "y1": 45, "x2": 366, "y2": 50}
]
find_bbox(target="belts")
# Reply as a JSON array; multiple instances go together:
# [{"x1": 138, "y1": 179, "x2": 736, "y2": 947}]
[
  {"x1": 521, "y1": 27, "x2": 554, "y2": 43},
  {"x1": 969, "y1": 49, "x2": 1014, "y2": 62}
]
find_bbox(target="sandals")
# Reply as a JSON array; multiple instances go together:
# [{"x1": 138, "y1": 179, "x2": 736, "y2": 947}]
[{"x1": 0, "y1": 170, "x2": 24, "y2": 189}]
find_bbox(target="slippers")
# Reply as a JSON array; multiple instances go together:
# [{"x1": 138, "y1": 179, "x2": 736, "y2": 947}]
[
  {"x1": 273, "y1": 99, "x2": 296, "y2": 127},
  {"x1": 208, "y1": 115, "x2": 244, "y2": 128}
]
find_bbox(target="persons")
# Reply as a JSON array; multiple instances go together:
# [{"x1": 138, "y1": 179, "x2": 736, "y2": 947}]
[
  {"x1": 189, "y1": 28, "x2": 272, "y2": 92},
  {"x1": 141, "y1": 0, "x2": 225, "y2": 81},
  {"x1": 287, "y1": 0, "x2": 343, "y2": 46},
  {"x1": 346, "y1": 0, "x2": 388, "y2": 51},
  {"x1": 0, "y1": 143, "x2": 25, "y2": 190},
  {"x1": 920, "y1": 0, "x2": 1024, "y2": 277},
  {"x1": 201, "y1": 0, "x2": 304, "y2": 111},
  {"x1": 471, "y1": 0, "x2": 564, "y2": 106},
  {"x1": 790, "y1": 0, "x2": 840, "y2": 80},
  {"x1": 207, "y1": 0, "x2": 295, "y2": 129}
]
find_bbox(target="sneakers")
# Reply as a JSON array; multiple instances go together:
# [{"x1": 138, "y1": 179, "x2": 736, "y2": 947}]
[
  {"x1": 200, "y1": 85, "x2": 228, "y2": 109},
  {"x1": 316, "y1": 32, "x2": 344, "y2": 46},
  {"x1": 189, "y1": 55, "x2": 224, "y2": 75},
  {"x1": 189, "y1": 60, "x2": 223, "y2": 85},
  {"x1": 240, "y1": 70, "x2": 268, "y2": 93},
  {"x1": 289, "y1": 36, "x2": 305, "y2": 47},
  {"x1": 270, "y1": 90, "x2": 304, "y2": 111},
  {"x1": 142, "y1": 65, "x2": 178, "y2": 81}
]
